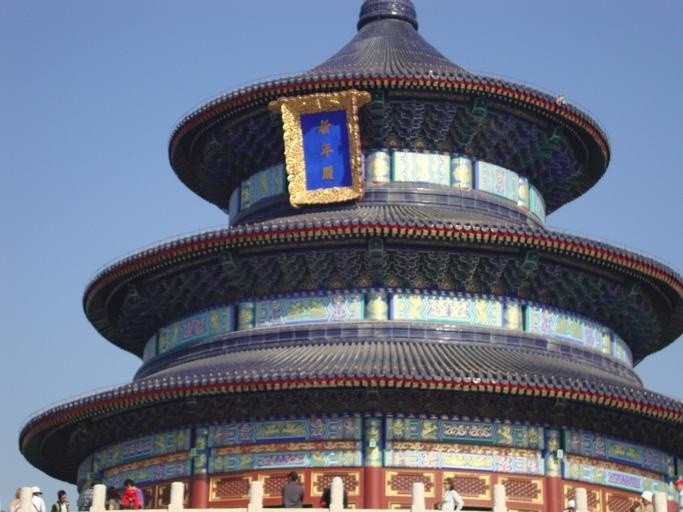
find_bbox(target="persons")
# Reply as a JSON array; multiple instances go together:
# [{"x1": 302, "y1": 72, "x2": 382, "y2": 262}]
[
  {"x1": 318, "y1": 479, "x2": 347, "y2": 509},
  {"x1": 626, "y1": 490, "x2": 655, "y2": 511},
  {"x1": 30, "y1": 485, "x2": 45, "y2": 512},
  {"x1": 123, "y1": 478, "x2": 143, "y2": 509},
  {"x1": 49, "y1": 489, "x2": 71, "y2": 512},
  {"x1": 671, "y1": 478, "x2": 683, "y2": 511},
  {"x1": 433, "y1": 476, "x2": 464, "y2": 512},
  {"x1": 565, "y1": 498, "x2": 575, "y2": 512},
  {"x1": 9, "y1": 487, "x2": 20, "y2": 512},
  {"x1": 105, "y1": 486, "x2": 121, "y2": 510},
  {"x1": 279, "y1": 470, "x2": 304, "y2": 508}
]
